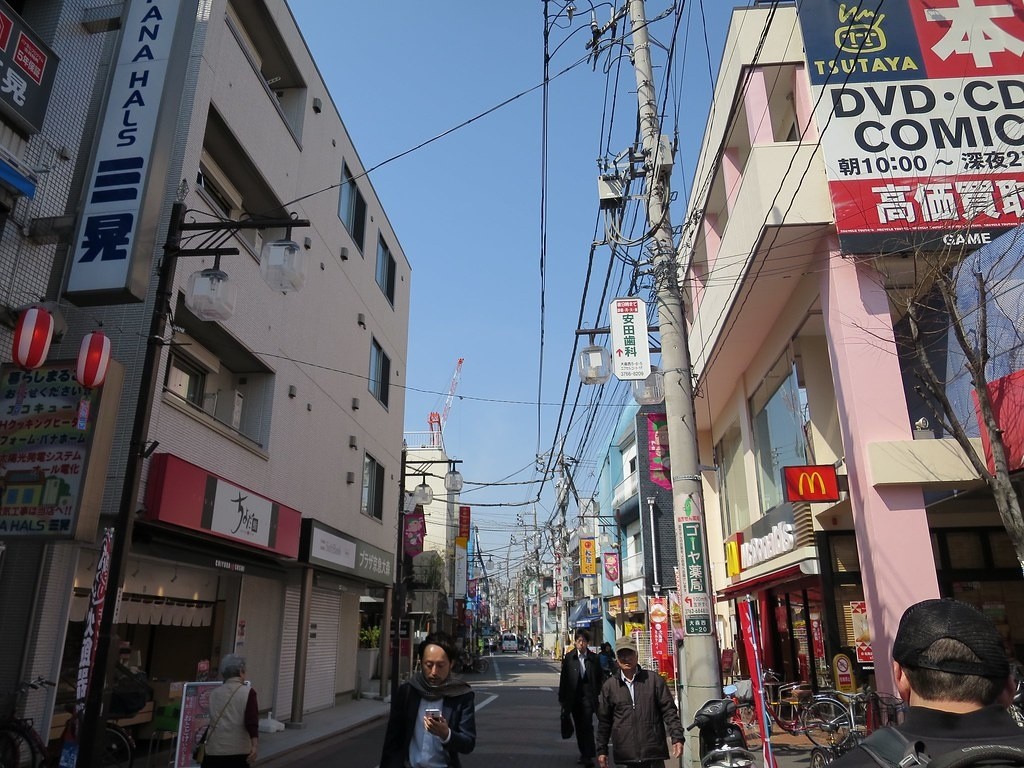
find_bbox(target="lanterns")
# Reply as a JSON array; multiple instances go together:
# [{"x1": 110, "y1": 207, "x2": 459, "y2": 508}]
[
  {"x1": 76, "y1": 328, "x2": 111, "y2": 389},
  {"x1": 12, "y1": 305, "x2": 54, "y2": 374}
]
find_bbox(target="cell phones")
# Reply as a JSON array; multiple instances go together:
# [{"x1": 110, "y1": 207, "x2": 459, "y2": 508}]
[{"x1": 425, "y1": 709, "x2": 440, "y2": 721}]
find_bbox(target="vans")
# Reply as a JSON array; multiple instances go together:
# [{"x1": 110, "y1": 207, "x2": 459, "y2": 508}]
[{"x1": 498, "y1": 633, "x2": 519, "y2": 653}]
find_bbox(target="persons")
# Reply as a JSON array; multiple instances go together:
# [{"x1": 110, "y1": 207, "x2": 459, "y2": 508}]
[
  {"x1": 379, "y1": 630, "x2": 476, "y2": 768},
  {"x1": 598, "y1": 643, "x2": 617, "y2": 682},
  {"x1": 557, "y1": 627, "x2": 605, "y2": 768},
  {"x1": 823, "y1": 599, "x2": 1024, "y2": 768},
  {"x1": 594, "y1": 635, "x2": 686, "y2": 768},
  {"x1": 487, "y1": 632, "x2": 543, "y2": 657},
  {"x1": 198, "y1": 660, "x2": 259, "y2": 768}
]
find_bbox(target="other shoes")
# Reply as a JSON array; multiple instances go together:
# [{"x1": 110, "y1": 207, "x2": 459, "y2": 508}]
[
  {"x1": 583, "y1": 758, "x2": 595, "y2": 768},
  {"x1": 577, "y1": 755, "x2": 584, "y2": 764}
]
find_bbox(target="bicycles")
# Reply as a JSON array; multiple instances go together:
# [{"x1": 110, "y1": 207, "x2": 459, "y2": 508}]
[
  {"x1": 0, "y1": 675, "x2": 137, "y2": 768},
  {"x1": 451, "y1": 646, "x2": 490, "y2": 674},
  {"x1": 739, "y1": 668, "x2": 852, "y2": 750}
]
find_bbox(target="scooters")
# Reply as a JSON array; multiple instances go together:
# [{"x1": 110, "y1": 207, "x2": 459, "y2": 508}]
[{"x1": 686, "y1": 684, "x2": 759, "y2": 768}]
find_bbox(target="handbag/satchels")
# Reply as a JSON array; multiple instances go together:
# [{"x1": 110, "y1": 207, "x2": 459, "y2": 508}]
[
  {"x1": 192, "y1": 743, "x2": 205, "y2": 763},
  {"x1": 560, "y1": 706, "x2": 574, "y2": 740}
]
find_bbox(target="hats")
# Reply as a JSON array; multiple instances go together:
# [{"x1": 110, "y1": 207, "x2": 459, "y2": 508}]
[
  {"x1": 892, "y1": 598, "x2": 1010, "y2": 679},
  {"x1": 615, "y1": 636, "x2": 637, "y2": 655}
]
